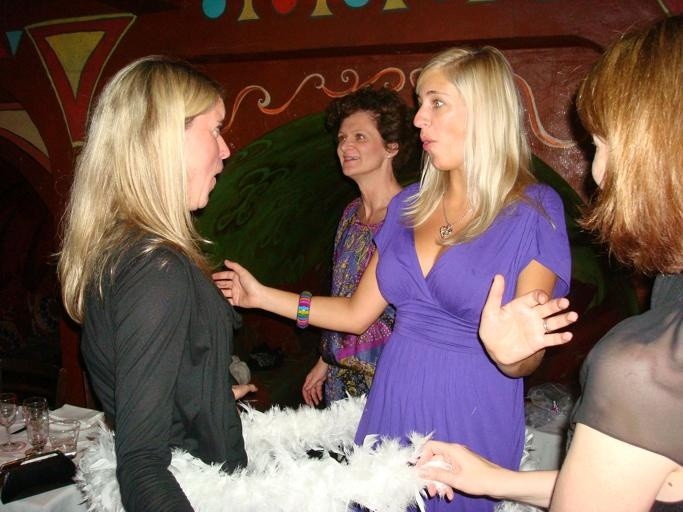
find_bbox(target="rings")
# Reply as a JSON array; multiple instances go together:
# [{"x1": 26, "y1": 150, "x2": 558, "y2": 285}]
[{"x1": 543, "y1": 317, "x2": 550, "y2": 333}]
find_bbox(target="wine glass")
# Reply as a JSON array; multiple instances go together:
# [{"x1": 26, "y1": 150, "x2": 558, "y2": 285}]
[
  {"x1": 0, "y1": 392, "x2": 20, "y2": 449},
  {"x1": 22, "y1": 397, "x2": 50, "y2": 457}
]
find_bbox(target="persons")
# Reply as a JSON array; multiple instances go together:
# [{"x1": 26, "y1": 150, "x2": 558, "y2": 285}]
[
  {"x1": 412, "y1": 13, "x2": 683, "y2": 512},
  {"x1": 300, "y1": 83, "x2": 413, "y2": 413},
  {"x1": 51, "y1": 54, "x2": 258, "y2": 512},
  {"x1": 208, "y1": 46, "x2": 582, "y2": 511}
]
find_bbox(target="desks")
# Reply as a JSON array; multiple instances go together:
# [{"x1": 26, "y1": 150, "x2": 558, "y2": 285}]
[{"x1": 0, "y1": 404, "x2": 117, "y2": 511}]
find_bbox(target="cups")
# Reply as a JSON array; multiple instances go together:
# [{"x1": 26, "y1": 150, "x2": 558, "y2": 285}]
[{"x1": 47, "y1": 418, "x2": 82, "y2": 459}]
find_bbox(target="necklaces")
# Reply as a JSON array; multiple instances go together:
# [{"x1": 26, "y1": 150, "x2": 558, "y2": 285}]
[{"x1": 437, "y1": 187, "x2": 473, "y2": 238}]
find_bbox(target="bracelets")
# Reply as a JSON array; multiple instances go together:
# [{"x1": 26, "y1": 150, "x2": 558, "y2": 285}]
[{"x1": 296, "y1": 291, "x2": 311, "y2": 330}]
